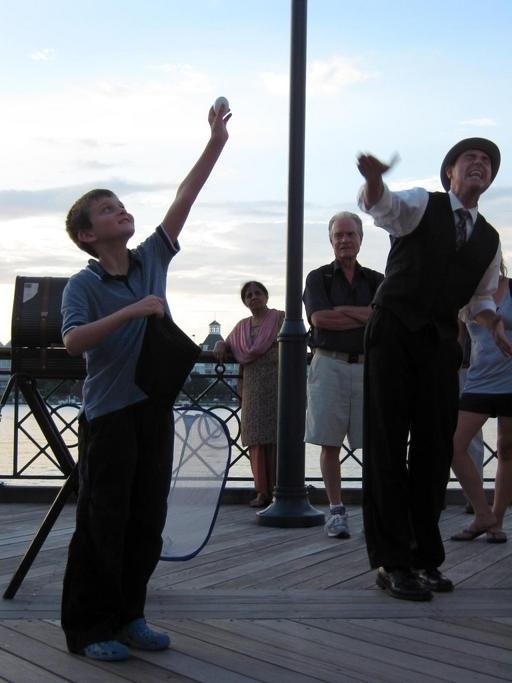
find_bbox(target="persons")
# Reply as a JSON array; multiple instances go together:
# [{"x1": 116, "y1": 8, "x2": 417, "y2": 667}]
[
  {"x1": 213, "y1": 282, "x2": 285, "y2": 508},
  {"x1": 61, "y1": 103, "x2": 231, "y2": 662},
  {"x1": 303, "y1": 212, "x2": 385, "y2": 539},
  {"x1": 355, "y1": 137, "x2": 512, "y2": 603},
  {"x1": 450, "y1": 248, "x2": 512, "y2": 545}
]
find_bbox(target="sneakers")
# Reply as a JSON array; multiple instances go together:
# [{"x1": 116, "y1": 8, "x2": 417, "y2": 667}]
[{"x1": 325, "y1": 505, "x2": 352, "y2": 539}]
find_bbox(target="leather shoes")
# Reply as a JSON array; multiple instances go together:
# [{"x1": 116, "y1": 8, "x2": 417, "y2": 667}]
[
  {"x1": 409, "y1": 565, "x2": 455, "y2": 593},
  {"x1": 374, "y1": 565, "x2": 435, "y2": 603}
]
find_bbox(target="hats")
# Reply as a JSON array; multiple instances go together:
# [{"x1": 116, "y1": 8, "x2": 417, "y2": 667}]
[{"x1": 440, "y1": 136, "x2": 501, "y2": 195}]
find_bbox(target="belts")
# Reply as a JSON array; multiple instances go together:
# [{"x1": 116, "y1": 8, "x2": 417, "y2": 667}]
[{"x1": 315, "y1": 347, "x2": 365, "y2": 364}]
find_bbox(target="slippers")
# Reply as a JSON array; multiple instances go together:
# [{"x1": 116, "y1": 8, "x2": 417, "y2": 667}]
[
  {"x1": 114, "y1": 617, "x2": 171, "y2": 652},
  {"x1": 83, "y1": 640, "x2": 130, "y2": 661},
  {"x1": 449, "y1": 519, "x2": 500, "y2": 541},
  {"x1": 486, "y1": 529, "x2": 507, "y2": 544}
]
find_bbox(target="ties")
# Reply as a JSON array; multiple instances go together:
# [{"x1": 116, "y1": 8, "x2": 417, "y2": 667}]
[{"x1": 454, "y1": 206, "x2": 471, "y2": 251}]
